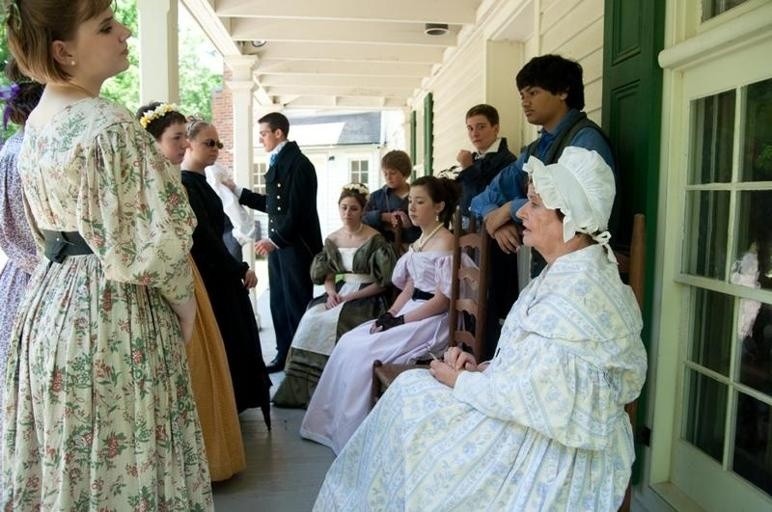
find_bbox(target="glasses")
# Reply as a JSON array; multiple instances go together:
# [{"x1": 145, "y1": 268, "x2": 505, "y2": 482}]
[{"x1": 202, "y1": 138, "x2": 223, "y2": 148}]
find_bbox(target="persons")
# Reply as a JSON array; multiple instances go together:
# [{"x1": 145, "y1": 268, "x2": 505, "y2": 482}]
[
  {"x1": 308, "y1": 145, "x2": 649, "y2": 512},
  {"x1": 468, "y1": 53, "x2": 622, "y2": 284},
  {"x1": 223, "y1": 112, "x2": 322, "y2": 376},
  {"x1": 181, "y1": 115, "x2": 274, "y2": 434},
  {"x1": 272, "y1": 181, "x2": 397, "y2": 411},
  {"x1": 361, "y1": 149, "x2": 422, "y2": 245},
  {"x1": 134, "y1": 101, "x2": 247, "y2": 483},
  {"x1": 0, "y1": 54, "x2": 49, "y2": 450},
  {"x1": 298, "y1": 175, "x2": 463, "y2": 458},
  {"x1": 456, "y1": 103, "x2": 518, "y2": 358},
  {"x1": 0, "y1": 0, "x2": 218, "y2": 510}
]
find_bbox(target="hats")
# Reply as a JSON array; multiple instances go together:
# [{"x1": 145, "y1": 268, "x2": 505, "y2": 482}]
[{"x1": 522, "y1": 145, "x2": 618, "y2": 264}]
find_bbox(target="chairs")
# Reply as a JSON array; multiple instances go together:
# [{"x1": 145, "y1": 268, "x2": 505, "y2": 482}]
[
  {"x1": 612, "y1": 213, "x2": 646, "y2": 510},
  {"x1": 371, "y1": 212, "x2": 491, "y2": 416}
]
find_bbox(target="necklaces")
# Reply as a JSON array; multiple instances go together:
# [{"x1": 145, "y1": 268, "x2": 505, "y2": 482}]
[{"x1": 418, "y1": 222, "x2": 445, "y2": 251}]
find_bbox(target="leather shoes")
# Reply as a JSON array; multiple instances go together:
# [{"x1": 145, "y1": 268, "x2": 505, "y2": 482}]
[{"x1": 266, "y1": 356, "x2": 284, "y2": 372}]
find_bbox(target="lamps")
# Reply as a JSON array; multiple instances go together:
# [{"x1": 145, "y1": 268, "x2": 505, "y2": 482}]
[{"x1": 425, "y1": 24, "x2": 448, "y2": 37}]
